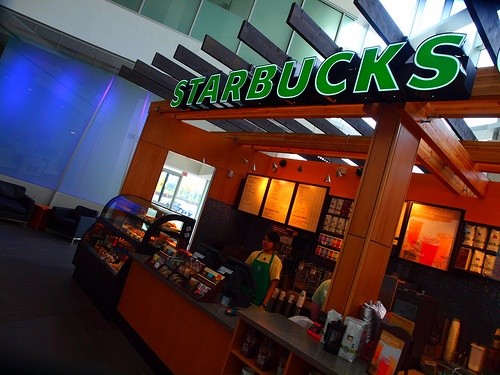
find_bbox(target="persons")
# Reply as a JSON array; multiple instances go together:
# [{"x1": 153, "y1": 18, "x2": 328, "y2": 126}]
[
  {"x1": 243, "y1": 232, "x2": 286, "y2": 312},
  {"x1": 310, "y1": 279, "x2": 332, "y2": 320}
]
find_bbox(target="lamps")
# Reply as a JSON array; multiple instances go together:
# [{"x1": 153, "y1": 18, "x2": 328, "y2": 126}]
[{"x1": 243, "y1": 122, "x2": 342, "y2": 183}]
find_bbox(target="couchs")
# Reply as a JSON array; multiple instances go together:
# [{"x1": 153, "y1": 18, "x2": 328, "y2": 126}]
[
  {"x1": 46, "y1": 204, "x2": 98, "y2": 244},
  {"x1": 0, "y1": 181, "x2": 35, "y2": 228}
]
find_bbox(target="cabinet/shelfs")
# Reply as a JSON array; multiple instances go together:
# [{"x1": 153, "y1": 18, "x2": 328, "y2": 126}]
[
  {"x1": 314, "y1": 194, "x2": 355, "y2": 261},
  {"x1": 453, "y1": 221, "x2": 500, "y2": 285},
  {"x1": 218, "y1": 318, "x2": 329, "y2": 375}
]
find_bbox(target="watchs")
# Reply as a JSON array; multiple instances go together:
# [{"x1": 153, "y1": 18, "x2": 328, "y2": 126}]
[{"x1": 262, "y1": 303, "x2": 267, "y2": 308}]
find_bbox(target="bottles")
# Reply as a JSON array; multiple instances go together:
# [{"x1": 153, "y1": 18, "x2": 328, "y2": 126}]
[
  {"x1": 240, "y1": 331, "x2": 260, "y2": 359},
  {"x1": 239, "y1": 367, "x2": 255, "y2": 375},
  {"x1": 256, "y1": 340, "x2": 276, "y2": 371}
]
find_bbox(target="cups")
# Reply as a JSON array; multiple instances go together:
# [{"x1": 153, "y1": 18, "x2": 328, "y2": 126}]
[
  {"x1": 419, "y1": 241, "x2": 439, "y2": 267},
  {"x1": 323, "y1": 319, "x2": 347, "y2": 356},
  {"x1": 407, "y1": 219, "x2": 423, "y2": 242}
]
